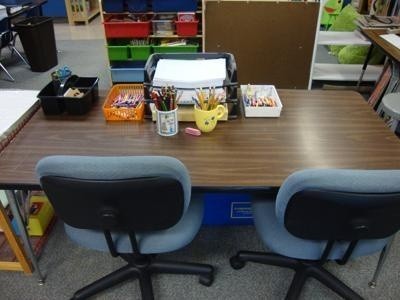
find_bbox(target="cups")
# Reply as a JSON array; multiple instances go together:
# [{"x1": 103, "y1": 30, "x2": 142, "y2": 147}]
[
  {"x1": 50, "y1": 70, "x2": 71, "y2": 94},
  {"x1": 193, "y1": 103, "x2": 225, "y2": 132},
  {"x1": 156, "y1": 105, "x2": 179, "y2": 136}
]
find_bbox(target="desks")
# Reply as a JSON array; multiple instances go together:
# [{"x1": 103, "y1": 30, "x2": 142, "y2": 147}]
[
  {"x1": 0, "y1": 0, "x2": 50, "y2": 57},
  {"x1": 353, "y1": 12, "x2": 400, "y2": 85},
  {"x1": 0, "y1": 88, "x2": 399, "y2": 288}
]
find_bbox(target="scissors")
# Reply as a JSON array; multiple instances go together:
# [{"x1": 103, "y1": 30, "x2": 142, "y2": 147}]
[{"x1": 56, "y1": 65, "x2": 72, "y2": 79}]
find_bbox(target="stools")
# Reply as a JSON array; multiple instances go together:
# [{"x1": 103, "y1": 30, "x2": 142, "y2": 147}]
[{"x1": 380, "y1": 90, "x2": 400, "y2": 133}]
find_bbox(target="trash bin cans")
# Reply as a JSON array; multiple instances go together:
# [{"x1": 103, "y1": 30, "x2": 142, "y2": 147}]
[{"x1": 14, "y1": 16, "x2": 57, "y2": 73}]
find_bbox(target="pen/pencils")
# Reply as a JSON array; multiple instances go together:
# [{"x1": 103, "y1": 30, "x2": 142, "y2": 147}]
[
  {"x1": 192, "y1": 84, "x2": 225, "y2": 109},
  {"x1": 149, "y1": 85, "x2": 184, "y2": 110},
  {"x1": 112, "y1": 94, "x2": 145, "y2": 118}
]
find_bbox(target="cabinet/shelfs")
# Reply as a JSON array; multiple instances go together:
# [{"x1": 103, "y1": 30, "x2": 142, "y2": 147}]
[
  {"x1": 99, "y1": 0, "x2": 204, "y2": 86},
  {"x1": 307, "y1": 0, "x2": 400, "y2": 92},
  {"x1": 65, "y1": 0, "x2": 100, "y2": 26}
]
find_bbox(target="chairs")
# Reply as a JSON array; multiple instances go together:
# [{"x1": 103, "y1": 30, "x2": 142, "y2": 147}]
[
  {"x1": 230, "y1": 162, "x2": 398, "y2": 300},
  {"x1": 0, "y1": 16, "x2": 31, "y2": 82},
  {"x1": 35, "y1": 152, "x2": 219, "y2": 300}
]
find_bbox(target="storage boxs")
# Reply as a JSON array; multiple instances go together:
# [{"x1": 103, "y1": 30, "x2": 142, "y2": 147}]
[
  {"x1": 102, "y1": 1, "x2": 200, "y2": 84},
  {"x1": 198, "y1": 190, "x2": 257, "y2": 227}
]
